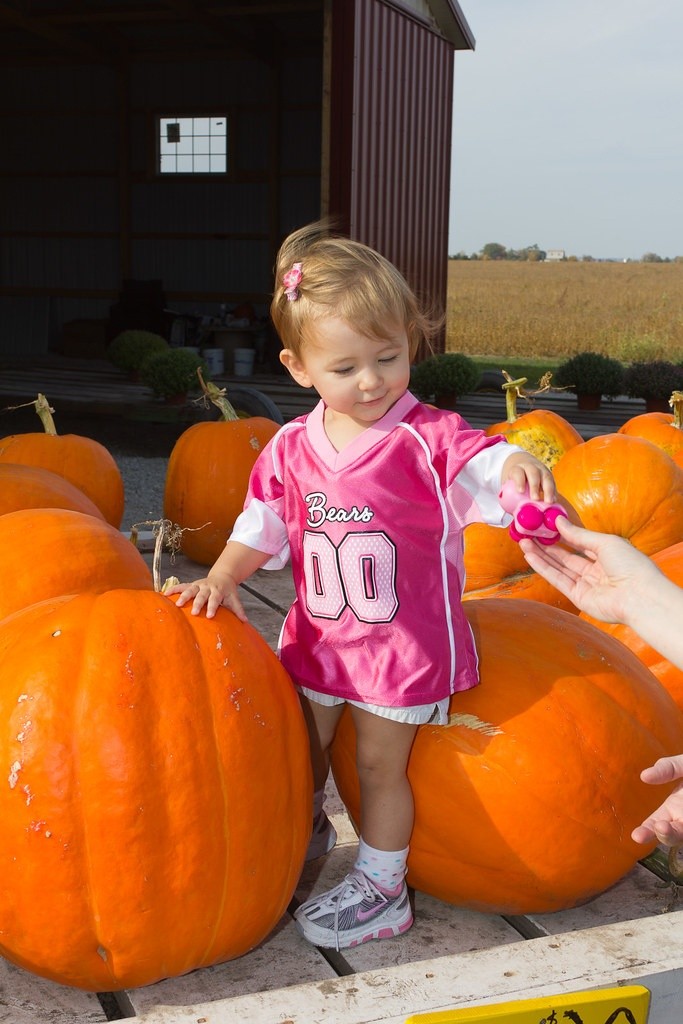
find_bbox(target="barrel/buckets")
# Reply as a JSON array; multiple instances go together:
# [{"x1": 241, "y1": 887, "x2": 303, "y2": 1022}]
[
  {"x1": 174, "y1": 346, "x2": 199, "y2": 353},
  {"x1": 233, "y1": 349, "x2": 256, "y2": 376},
  {"x1": 204, "y1": 348, "x2": 225, "y2": 377}
]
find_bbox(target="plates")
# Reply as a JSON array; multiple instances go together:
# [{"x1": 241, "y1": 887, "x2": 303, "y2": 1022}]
[{"x1": 121, "y1": 532, "x2": 156, "y2": 549}]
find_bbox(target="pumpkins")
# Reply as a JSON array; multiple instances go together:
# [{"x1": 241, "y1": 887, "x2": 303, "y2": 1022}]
[{"x1": 0, "y1": 373, "x2": 683, "y2": 991}]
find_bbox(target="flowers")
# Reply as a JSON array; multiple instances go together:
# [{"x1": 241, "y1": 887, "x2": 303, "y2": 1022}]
[{"x1": 283, "y1": 269, "x2": 303, "y2": 287}]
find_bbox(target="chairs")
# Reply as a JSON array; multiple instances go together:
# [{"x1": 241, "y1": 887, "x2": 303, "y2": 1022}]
[{"x1": 110, "y1": 278, "x2": 168, "y2": 334}]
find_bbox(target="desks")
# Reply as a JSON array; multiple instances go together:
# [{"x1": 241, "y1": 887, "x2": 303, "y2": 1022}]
[{"x1": 203, "y1": 323, "x2": 262, "y2": 371}]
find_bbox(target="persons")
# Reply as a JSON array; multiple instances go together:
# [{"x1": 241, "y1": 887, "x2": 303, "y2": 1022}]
[
  {"x1": 160, "y1": 224, "x2": 558, "y2": 951},
  {"x1": 520, "y1": 514, "x2": 683, "y2": 847}
]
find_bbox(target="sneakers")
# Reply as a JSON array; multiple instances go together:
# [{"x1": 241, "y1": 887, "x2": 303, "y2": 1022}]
[
  {"x1": 304, "y1": 808, "x2": 337, "y2": 862},
  {"x1": 292, "y1": 866, "x2": 413, "y2": 952}
]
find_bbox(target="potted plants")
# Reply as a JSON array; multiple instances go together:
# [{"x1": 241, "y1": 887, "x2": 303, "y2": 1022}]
[
  {"x1": 410, "y1": 352, "x2": 482, "y2": 410},
  {"x1": 108, "y1": 330, "x2": 210, "y2": 405},
  {"x1": 554, "y1": 350, "x2": 683, "y2": 412}
]
